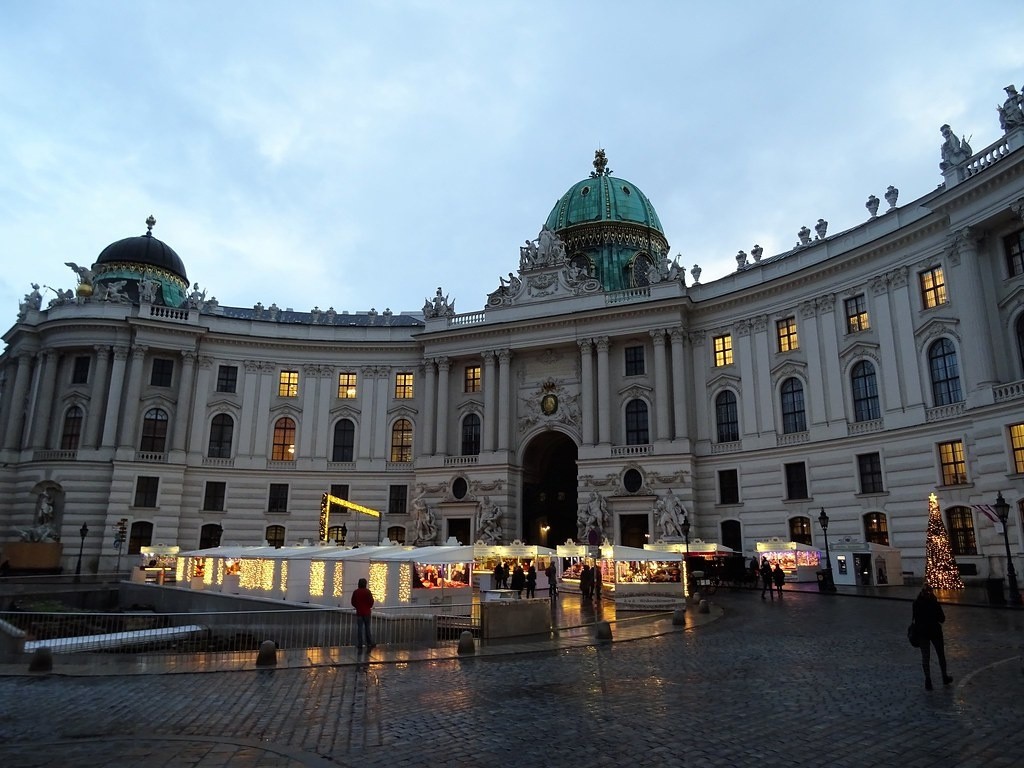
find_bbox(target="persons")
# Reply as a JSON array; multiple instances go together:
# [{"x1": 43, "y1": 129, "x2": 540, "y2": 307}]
[
  {"x1": 773, "y1": 564, "x2": 785, "y2": 595},
  {"x1": 520, "y1": 224, "x2": 557, "y2": 263},
  {"x1": 749, "y1": 556, "x2": 773, "y2": 599},
  {"x1": 351, "y1": 579, "x2": 376, "y2": 647},
  {"x1": 502, "y1": 273, "x2": 519, "y2": 293},
  {"x1": 187, "y1": 283, "x2": 205, "y2": 307},
  {"x1": 495, "y1": 561, "x2": 558, "y2": 599},
  {"x1": 416, "y1": 502, "x2": 435, "y2": 540},
  {"x1": 912, "y1": 582, "x2": 953, "y2": 690},
  {"x1": 478, "y1": 495, "x2": 501, "y2": 540},
  {"x1": 582, "y1": 493, "x2": 603, "y2": 538},
  {"x1": 580, "y1": 565, "x2": 602, "y2": 603},
  {"x1": 658, "y1": 490, "x2": 688, "y2": 540}
]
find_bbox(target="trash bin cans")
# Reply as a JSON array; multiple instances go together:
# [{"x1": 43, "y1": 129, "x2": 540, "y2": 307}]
[
  {"x1": 814, "y1": 568, "x2": 833, "y2": 592},
  {"x1": 985, "y1": 578, "x2": 1008, "y2": 603}
]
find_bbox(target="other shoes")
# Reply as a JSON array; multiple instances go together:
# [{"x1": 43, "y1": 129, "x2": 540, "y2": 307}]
[
  {"x1": 778, "y1": 593, "x2": 783, "y2": 594},
  {"x1": 771, "y1": 594, "x2": 773, "y2": 598},
  {"x1": 549, "y1": 593, "x2": 559, "y2": 595},
  {"x1": 357, "y1": 641, "x2": 362, "y2": 648},
  {"x1": 367, "y1": 642, "x2": 371, "y2": 647},
  {"x1": 581, "y1": 596, "x2": 602, "y2": 601},
  {"x1": 761, "y1": 594, "x2": 766, "y2": 599}
]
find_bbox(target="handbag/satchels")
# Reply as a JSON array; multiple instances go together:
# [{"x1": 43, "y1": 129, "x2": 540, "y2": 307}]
[{"x1": 907, "y1": 622, "x2": 921, "y2": 647}]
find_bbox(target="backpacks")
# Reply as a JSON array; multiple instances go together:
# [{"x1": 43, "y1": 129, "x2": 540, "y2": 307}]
[{"x1": 545, "y1": 566, "x2": 555, "y2": 577}]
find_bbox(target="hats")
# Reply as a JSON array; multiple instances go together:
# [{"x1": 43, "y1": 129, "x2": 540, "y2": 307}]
[
  {"x1": 498, "y1": 562, "x2": 501, "y2": 564},
  {"x1": 764, "y1": 561, "x2": 769, "y2": 565},
  {"x1": 775, "y1": 563, "x2": 779, "y2": 567}
]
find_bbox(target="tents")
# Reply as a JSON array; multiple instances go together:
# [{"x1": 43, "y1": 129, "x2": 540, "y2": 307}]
[
  {"x1": 557, "y1": 540, "x2": 599, "y2": 593},
  {"x1": 644, "y1": 539, "x2": 732, "y2": 587},
  {"x1": 601, "y1": 538, "x2": 615, "y2": 601},
  {"x1": 756, "y1": 537, "x2": 820, "y2": 583},
  {"x1": 501, "y1": 540, "x2": 562, "y2": 590},
  {"x1": 473, "y1": 541, "x2": 502, "y2": 586},
  {"x1": 613, "y1": 544, "x2": 684, "y2": 611},
  {"x1": 175, "y1": 545, "x2": 473, "y2": 617}
]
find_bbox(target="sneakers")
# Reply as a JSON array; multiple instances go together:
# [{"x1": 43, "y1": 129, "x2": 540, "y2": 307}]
[
  {"x1": 925, "y1": 679, "x2": 933, "y2": 690},
  {"x1": 942, "y1": 675, "x2": 953, "y2": 684}
]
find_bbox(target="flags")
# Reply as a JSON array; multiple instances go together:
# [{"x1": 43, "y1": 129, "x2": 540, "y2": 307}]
[{"x1": 972, "y1": 505, "x2": 1001, "y2": 522}]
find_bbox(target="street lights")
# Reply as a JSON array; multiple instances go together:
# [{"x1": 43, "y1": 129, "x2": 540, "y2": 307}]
[
  {"x1": 991, "y1": 490, "x2": 1021, "y2": 598},
  {"x1": 680, "y1": 517, "x2": 691, "y2": 555},
  {"x1": 818, "y1": 506, "x2": 832, "y2": 568},
  {"x1": 73, "y1": 521, "x2": 89, "y2": 582},
  {"x1": 341, "y1": 523, "x2": 348, "y2": 546}
]
